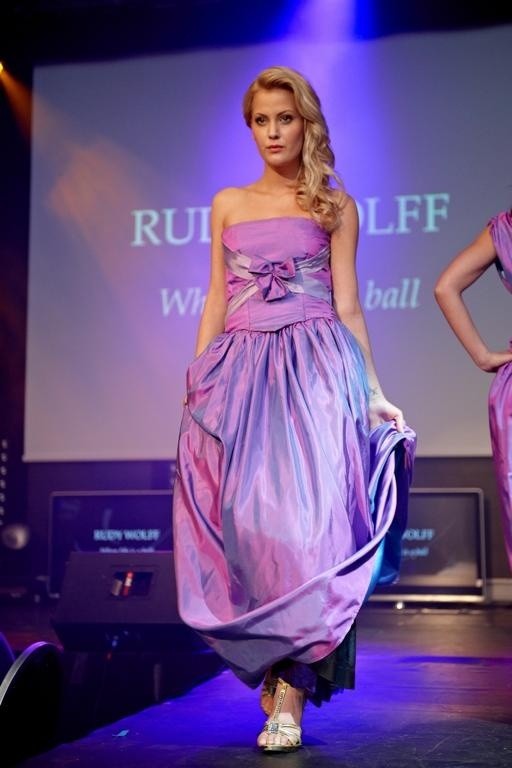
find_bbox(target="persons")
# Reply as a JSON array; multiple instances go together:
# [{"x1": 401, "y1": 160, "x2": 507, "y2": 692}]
[
  {"x1": 171, "y1": 65, "x2": 418, "y2": 752},
  {"x1": 433, "y1": 207, "x2": 511, "y2": 581}
]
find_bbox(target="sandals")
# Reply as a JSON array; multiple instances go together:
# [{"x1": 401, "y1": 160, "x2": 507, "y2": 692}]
[{"x1": 261, "y1": 668, "x2": 302, "y2": 753}]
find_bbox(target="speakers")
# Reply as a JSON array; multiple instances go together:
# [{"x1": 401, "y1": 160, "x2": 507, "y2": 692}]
[{"x1": 51, "y1": 551, "x2": 182, "y2": 646}]
